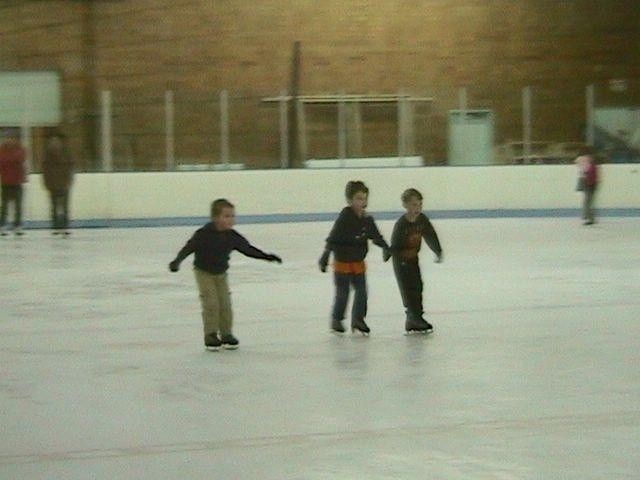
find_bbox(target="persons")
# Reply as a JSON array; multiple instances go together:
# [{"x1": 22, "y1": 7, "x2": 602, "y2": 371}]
[
  {"x1": 41, "y1": 131, "x2": 75, "y2": 239},
  {"x1": 0, "y1": 129, "x2": 28, "y2": 237},
  {"x1": 390, "y1": 188, "x2": 442, "y2": 335},
  {"x1": 573, "y1": 150, "x2": 597, "y2": 226},
  {"x1": 168, "y1": 198, "x2": 283, "y2": 352},
  {"x1": 318, "y1": 181, "x2": 391, "y2": 336}
]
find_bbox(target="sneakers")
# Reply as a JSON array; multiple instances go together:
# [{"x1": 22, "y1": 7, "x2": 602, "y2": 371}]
[
  {"x1": 405, "y1": 311, "x2": 432, "y2": 331},
  {"x1": 221, "y1": 335, "x2": 238, "y2": 345},
  {"x1": 331, "y1": 320, "x2": 344, "y2": 332},
  {"x1": 0, "y1": 227, "x2": 71, "y2": 235},
  {"x1": 204, "y1": 334, "x2": 221, "y2": 346},
  {"x1": 352, "y1": 316, "x2": 370, "y2": 333}
]
